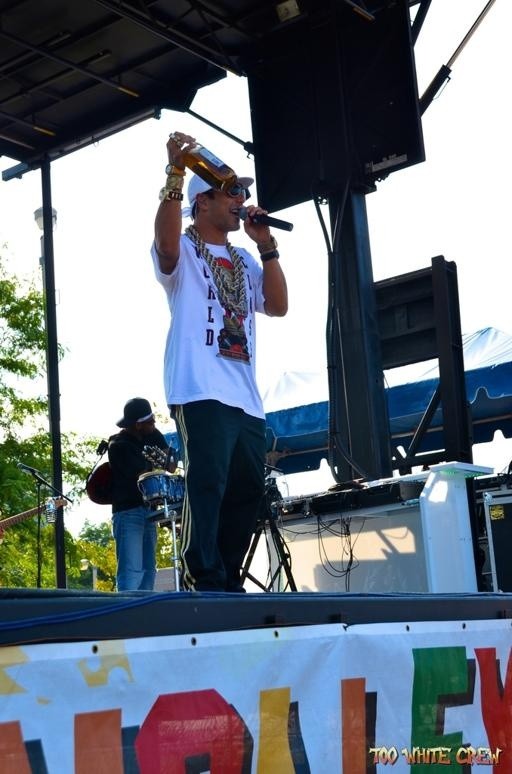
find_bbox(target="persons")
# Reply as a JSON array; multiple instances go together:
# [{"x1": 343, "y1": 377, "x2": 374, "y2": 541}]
[
  {"x1": 146, "y1": 129, "x2": 289, "y2": 594},
  {"x1": 102, "y1": 396, "x2": 180, "y2": 592}
]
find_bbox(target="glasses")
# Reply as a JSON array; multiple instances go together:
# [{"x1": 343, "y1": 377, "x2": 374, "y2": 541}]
[{"x1": 227, "y1": 183, "x2": 251, "y2": 200}]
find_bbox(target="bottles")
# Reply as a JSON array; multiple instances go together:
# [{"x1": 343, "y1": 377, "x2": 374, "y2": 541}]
[
  {"x1": 44, "y1": 490, "x2": 59, "y2": 524},
  {"x1": 169, "y1": 131, "x2": 236, "y2": 194}
]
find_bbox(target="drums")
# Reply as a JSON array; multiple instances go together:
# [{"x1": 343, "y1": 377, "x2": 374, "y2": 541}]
[
  {"x1": 137, "y1": 469, "x2": 176, "y2": 506},
  {"x1": 164, "y1": 475, "x2": 185, "y2": 503}
]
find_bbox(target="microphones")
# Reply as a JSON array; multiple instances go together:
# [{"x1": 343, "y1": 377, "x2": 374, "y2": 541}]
[
  {"x1": 16, "y1": 462, "x2": 40, "y2": 474},
  {"x1": 238, "y1": 206, "x2": 294, "y2": 234}
]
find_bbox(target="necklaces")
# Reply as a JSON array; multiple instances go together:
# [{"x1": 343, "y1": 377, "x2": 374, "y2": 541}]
[
  {"x1": 184, "y1": 227, "x2": 249, "y2": 318},
  {"x1": 189, "y1": 224, "x2": 240, "y2": 293}
]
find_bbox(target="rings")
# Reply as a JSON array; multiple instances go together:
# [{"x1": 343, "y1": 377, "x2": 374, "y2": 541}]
[
  {"x1": 176, "y1": 141, "x2": 183, "y2": 147},
  {"x1": 174, "y1": 136, "x2": 179, "y2": 142},
  {"x1": 168, "y1": 132, "x2": 175, "y2": 139}
]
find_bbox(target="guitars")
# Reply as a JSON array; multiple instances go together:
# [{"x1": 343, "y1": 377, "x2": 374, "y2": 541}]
[
  {"x1": 86, "y1": 461, "x2": 146, "y2": 504},
  {"x1": 0, "y1": 495, "x2": 67, "y2": 546}
]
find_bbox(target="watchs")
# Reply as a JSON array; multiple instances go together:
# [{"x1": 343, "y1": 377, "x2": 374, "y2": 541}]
[
  {"x1": 164, "y1": 163, "x2": 187, "y2": 177},
  {"x1": 158, "y1": 186, "x2": 184, "y2": 202},
  {"x1": 166, "y1": 173, "x2": 184, "y2": 189}
]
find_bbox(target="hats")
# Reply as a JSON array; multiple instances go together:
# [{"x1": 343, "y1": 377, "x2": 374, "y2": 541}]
[
  {"x1": 187, "y1": 173, "x2": 254, "y2": 205},
  {"x1": 116, "y1": 398, "x2": 153, "y2": 428}
]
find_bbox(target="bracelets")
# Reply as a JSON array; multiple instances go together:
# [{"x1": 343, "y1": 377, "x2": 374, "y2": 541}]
[
  {"x1": 257, "y1": 236, "x2": 278, "y2": 253},
  {"x1": 260, "y1": 249, "x2": 279, "y2": 261}
]
